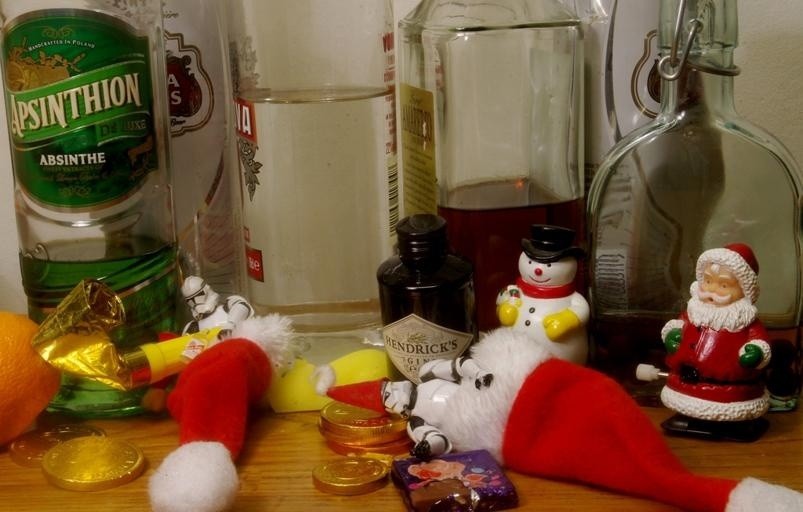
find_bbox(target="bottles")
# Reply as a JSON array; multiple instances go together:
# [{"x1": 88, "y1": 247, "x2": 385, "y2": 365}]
[
  {"x1": 587, "y1": 0, "x2": 803, "y2": 414},
  {"x1": 391, "y1": 2, "x2": 588, "y2": 332},
  {"x1": 234, "y1": 0, "x2": 393, "y2": 324},
  {"x1": 0, "y1": 2, "x2": 256, "y2": 424},
  {"x1": 370, "y1": 216, "x2": 481, "y2": 393}
]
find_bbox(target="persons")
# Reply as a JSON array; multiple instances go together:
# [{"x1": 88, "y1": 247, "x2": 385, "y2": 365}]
[
  {"x1": 380, "y1": 349, "x2": 491, "y2": 458},
  {"x1": 634, "y1": 242, "x2": 774, "y2": 441},
  {"x1": 176, "y1": 274, "x2": 254, "y2": 337}
]
